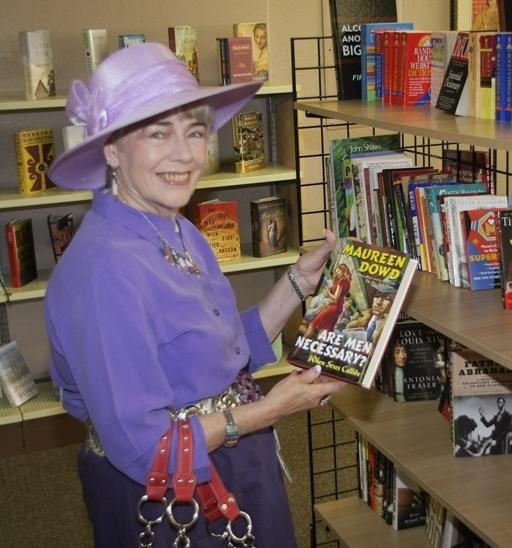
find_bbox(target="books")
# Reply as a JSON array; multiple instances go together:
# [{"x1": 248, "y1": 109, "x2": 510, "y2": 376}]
[
  {"x1": 232, "y1": 112, "x2": 264, "y2": 173},
  {"x1": 250, "y1": 195, "x2": 287, "y2": 257},
  {"x1": 62, "y1": 125, "x2": 84, "y2": 152},
  {"x1": 355, "y1": 431, "x2": 486, "y2": 547},
  {"x1": 5, "y1": 217, "x2": 37, "y2": 287},
  {"x1": 200, "y1": 132, "x2": 220, "y2": 175},
  {"x1": 118, "y1": 33, "x2": 145, "y2": 50},
  {"x1": 82, "y1": 29, "x2": 107, "y2": 81},
  {"x1": 216, "y1": 22, "x2": 270, "y2": 86},
  {"x1": 48, "y1": 211, "x2": 75, "y2": 263},
  {"x1": 287, "y1": 241, "x2": 418, "y2": 390},
  {"x1": 372, "y1": 311, "x2": 511, "y2": 457},
  {"x1": 329, "y1": 0, "x2": 512, "y2": 123},
  {"x1": 192, "y1": 198, "x2": 241, "y2": 262},
  {"x1": 21, "y1": 31, "x2": 55, "y2": 100},
  {"x1": 15, "y1": 128, "x2": 55, "y2": 193},
  {"x1": 0, "y1": 342, "x2": 38, "y2": 407},
  {"x1": 330, "y1": 133, "x2": 512, "y2": 310},
  {"x1": 168, "y1": 26, "x2": 199, "y2": 86}
]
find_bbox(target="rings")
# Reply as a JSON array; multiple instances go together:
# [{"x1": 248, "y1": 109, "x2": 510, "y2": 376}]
[{"x1": 321, "y1": 395, "x2": 331, "y2": 406}]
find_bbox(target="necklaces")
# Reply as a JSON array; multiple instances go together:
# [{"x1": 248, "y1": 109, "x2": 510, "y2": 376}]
[{"x1": 118, "y1": 190, "x2": 200, "y2": 278}]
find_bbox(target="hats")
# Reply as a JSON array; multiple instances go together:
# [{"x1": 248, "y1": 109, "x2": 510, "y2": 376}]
[
  {"x1": 366, "y1": 275, "x2": 400, "y2": 293},
  {"x1": 48, "y1": 42, "x2": 264, "y2": 191}
]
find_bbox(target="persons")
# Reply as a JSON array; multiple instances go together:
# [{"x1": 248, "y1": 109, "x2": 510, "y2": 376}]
[{"x1": 48, "y1": 44, "x2": 351, "y2": 548}]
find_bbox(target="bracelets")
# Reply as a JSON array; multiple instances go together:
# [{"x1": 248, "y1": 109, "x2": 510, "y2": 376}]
[
  {"x1": 224, "y1": 408, "x2": 240, "y2": 448},
  {"x1": 287, "y1": 266, "x2": 305, "y2": 301}
]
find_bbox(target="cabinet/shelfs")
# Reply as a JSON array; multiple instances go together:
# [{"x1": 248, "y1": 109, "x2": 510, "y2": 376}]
[
  {"x1": 0, "y1": 80, "x2": 304, "y2": 426},
  {"x1": 290, "y1": 35, "x2": 512, "y2": 548}
]
